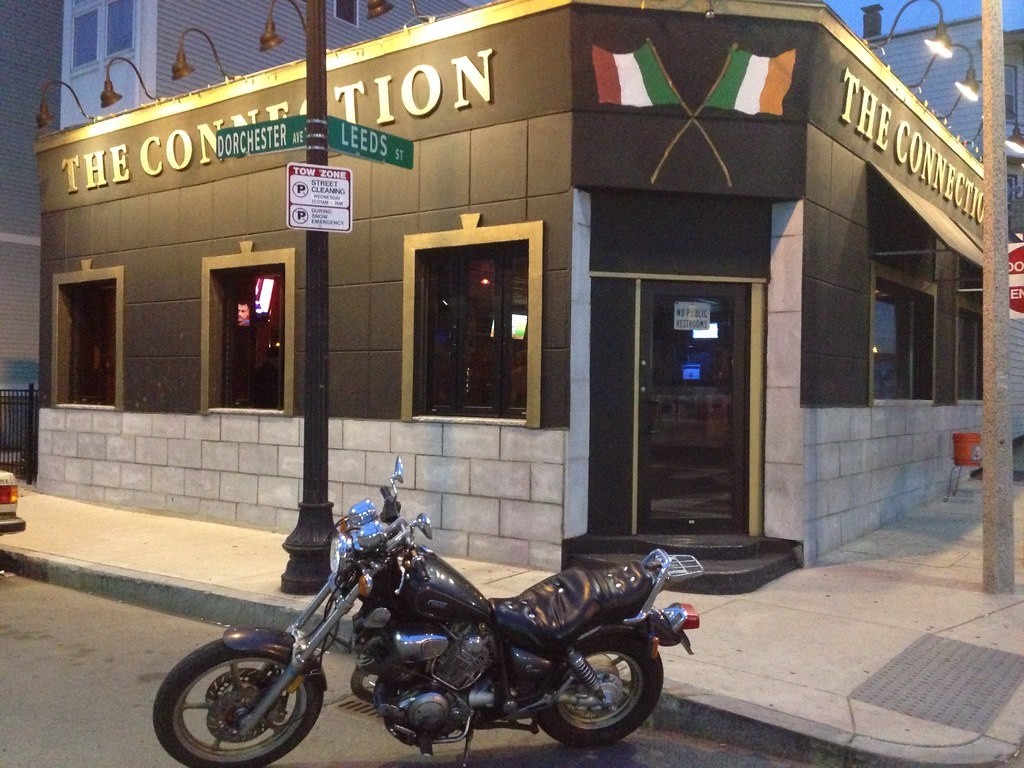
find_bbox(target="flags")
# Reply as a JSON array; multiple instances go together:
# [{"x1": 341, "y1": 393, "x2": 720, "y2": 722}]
[
  {"x1": 705, "y1": 46, "x2": 796, "y2": 116},
  {"x1": 591, "y1": 40, "x2": 680, "y2": 107}
]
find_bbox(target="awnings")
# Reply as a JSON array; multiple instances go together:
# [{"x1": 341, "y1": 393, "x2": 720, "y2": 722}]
[
  {"x1": 866, "y1": 159, "x2": 984, "y2": 267},
  {"x1": 952, "y1": 218, "x2": 984, "y2": 253}
]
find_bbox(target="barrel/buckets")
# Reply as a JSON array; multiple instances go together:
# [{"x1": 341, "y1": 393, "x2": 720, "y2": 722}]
[{"x1": 952, "y1": 431, "x2": 982, "y2": 466}]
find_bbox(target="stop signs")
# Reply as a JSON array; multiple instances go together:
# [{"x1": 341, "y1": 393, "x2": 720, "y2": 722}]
[{"x1": 1005, "y1": 243, "x2": 1024, "y2": 315}]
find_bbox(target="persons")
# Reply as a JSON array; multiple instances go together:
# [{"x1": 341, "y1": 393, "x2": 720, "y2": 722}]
[{"x1": 237, "y1": 304, "x2": 250, "y2": 326}]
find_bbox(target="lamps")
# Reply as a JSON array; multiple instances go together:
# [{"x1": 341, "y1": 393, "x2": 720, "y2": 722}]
[
  {"x1": 962, "y1": 107, "x2": 1024, "y2": 154},
  {"x1": 259, "y1": 0, "x2": 306, "y2": 52},
  {"x1": 37, "y1": 81, "x2": 95, "y2": 129},
  {"x1": 367, "y1": 0, "x2": 428, "y2": 22},
  {"x1": 101, "y1": 57, "x2": 160, "y2": 108},
  {"x1": 868, "y1": 0, "x2": 952, "y2": 59},
  {"x1": 172, "y1": 28, "x2": 234, "y2": 80},
  {"x1": 907, "y1": 43, "x2": 978, "y2": 101}
]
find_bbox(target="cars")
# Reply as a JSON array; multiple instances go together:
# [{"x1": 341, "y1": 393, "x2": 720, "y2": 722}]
[{"x1": 0, "y1": 469, "x2": 24, "y2": 538}]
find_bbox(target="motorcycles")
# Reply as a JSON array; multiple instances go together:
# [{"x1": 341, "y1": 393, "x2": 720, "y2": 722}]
[{"x1": 152, "y1": 454, "x2": 706, "y2": 767}]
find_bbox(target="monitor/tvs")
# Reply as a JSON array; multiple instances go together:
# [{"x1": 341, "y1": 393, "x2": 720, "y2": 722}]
[
  {"x1": 487, "y1": 310, "x2": 528, "y2": 341},
  {"x1": 693, "y1": 323, "x2": 720, "y2": 339},
  {"x1": 252, "y1": 277, "x2": 277, "y2": 320}
]
